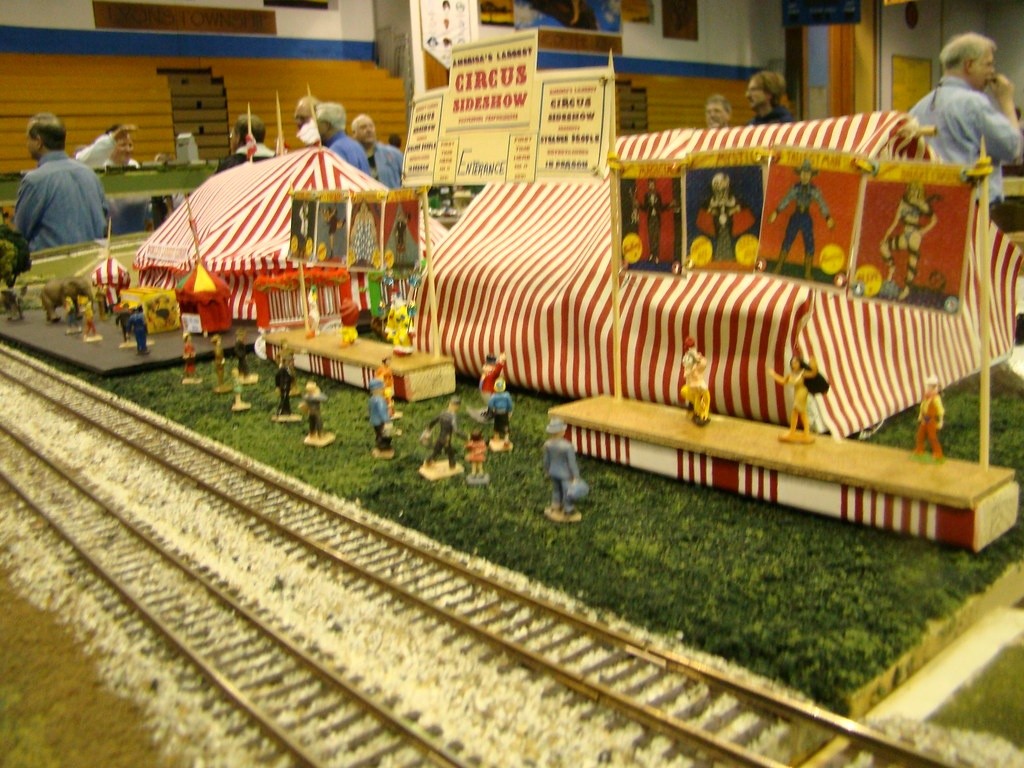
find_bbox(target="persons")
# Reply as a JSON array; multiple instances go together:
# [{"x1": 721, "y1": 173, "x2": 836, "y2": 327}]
[
  {"x1": 743, "y1": 70, "x2": 798, "y2": 124},
  {"x1": 913, "y1": 376, "x2": 945, "y2": 459},
  {"x1": 541, "y1": 418, "x2": 580, "y2": 516},
  {"x1": 908, "y1": 32, "x2": 1021, "y2": 209},
  {"x1": 706, "y1": 94, "x2": 730, "y2": 129},
  {"x1": 76, "y1": 123, "x2": 148, "y2": 235},
  {"x1": 385, "y1": 289, "x2": 416, "y2": 357},
  {"x1": 64, "y1": 294, "x2": 148, "y2": 356},
  {"x1": 179, "y1": 329, "x2": 328, "y2": 439},
  {"x1": 678, "y1": 349, "x2": 710, "y2": 422},
  {"x1": 295, "y1": 95, "x2": 404, "y2": 187},
  {"x1": 767, "y1": 355, "x2": 829, "y2": 437},
  {"x1": 15, "y1": 110, "x2": 109, "y2": 252},
  {"x1": 215, "y1": 114, "x2": 276, "y2": 175},
  {"x1": 427, "y1": 351, "x2": 513, "y2": 479},
  {"x1": 369, "y1": 353, "x2": 395, "y2": 453}
]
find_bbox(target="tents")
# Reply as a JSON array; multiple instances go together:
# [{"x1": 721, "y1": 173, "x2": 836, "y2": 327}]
[
  {"x1": 132, "y1": 147, "x2": 449, "y2": 323},
  {"x1": 407, "y1": 109, "x2": 1024, "y2": 438}
]
